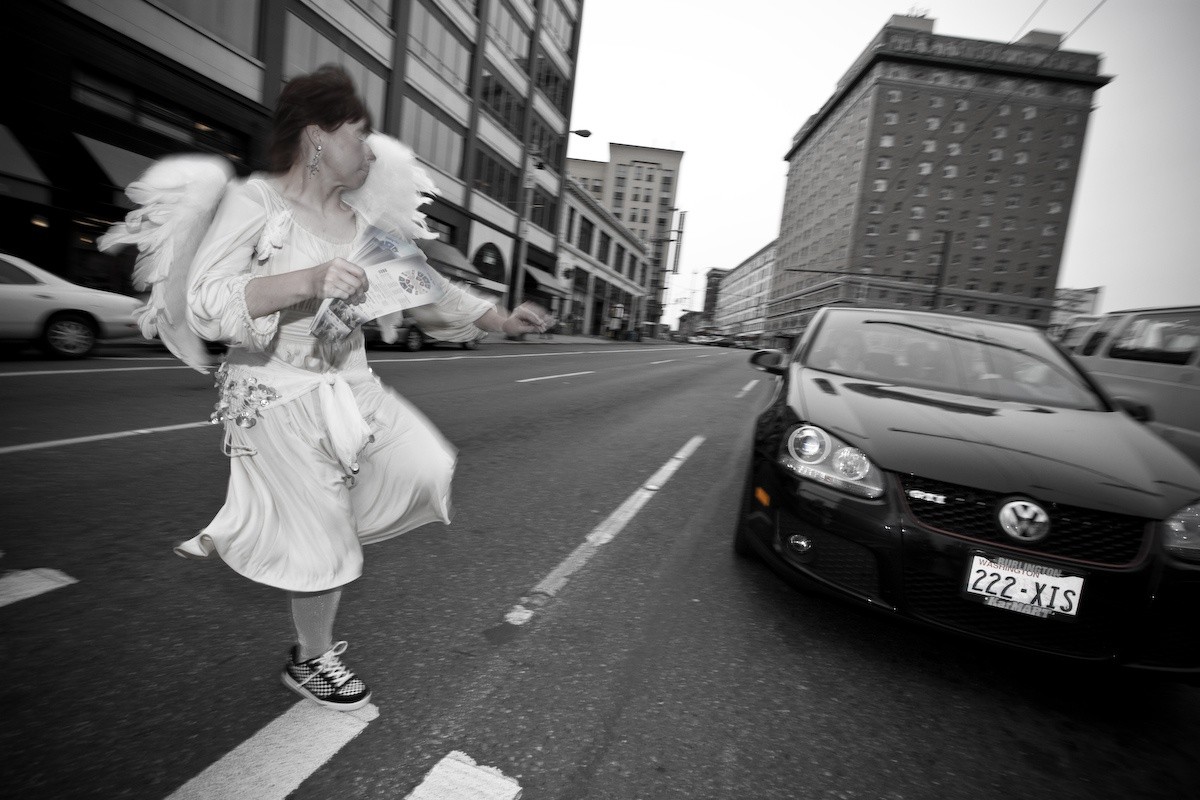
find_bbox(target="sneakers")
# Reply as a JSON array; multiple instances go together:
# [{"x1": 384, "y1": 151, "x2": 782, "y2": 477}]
[{"x1": 279, "y1": 641, "x2": 372, "y2": 712}]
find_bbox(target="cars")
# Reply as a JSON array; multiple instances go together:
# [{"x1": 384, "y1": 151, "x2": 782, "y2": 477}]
[
  {"x1": 1, "y1": 252, "x2": 150, "y2": 361},
  {"x1": 735, "y1": 309, "x2": 1200, "y2": 685}
]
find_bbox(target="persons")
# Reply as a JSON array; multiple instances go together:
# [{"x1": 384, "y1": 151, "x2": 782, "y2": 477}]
[{"x1": 95, "y1": 60, "x2": 546, "y2": 715}]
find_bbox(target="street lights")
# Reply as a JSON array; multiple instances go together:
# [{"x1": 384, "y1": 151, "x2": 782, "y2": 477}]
[{"x1": 506, "y1": 129, "x2": 593, "y2": 308}]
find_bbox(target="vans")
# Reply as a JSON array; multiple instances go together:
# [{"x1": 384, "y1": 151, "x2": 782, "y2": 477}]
[{"x1": 1056, "y1": 305, "x2": 1200, "y2": 457}]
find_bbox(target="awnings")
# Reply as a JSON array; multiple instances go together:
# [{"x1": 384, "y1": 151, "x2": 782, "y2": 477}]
[{"x1": 408, "y1": 234, "x2": 569, "y2": 299}]
